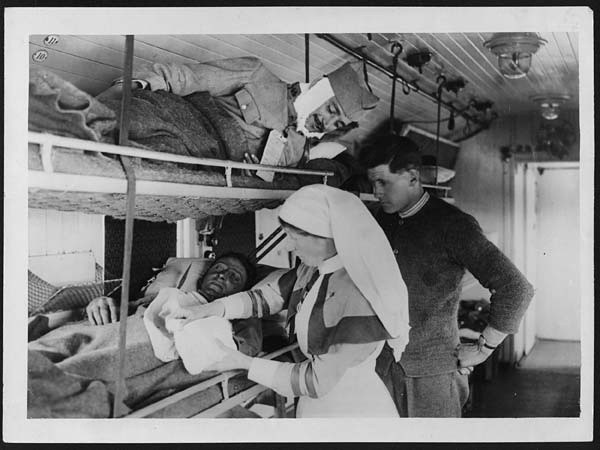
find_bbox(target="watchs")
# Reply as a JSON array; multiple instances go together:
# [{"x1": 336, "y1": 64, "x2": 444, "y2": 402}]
[{"x1": 475, "y1": 333, "x2": 499, "y2": 352}]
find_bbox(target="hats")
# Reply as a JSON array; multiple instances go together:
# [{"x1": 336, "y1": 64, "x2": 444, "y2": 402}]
[{"x1": 327, "y1": 61, "x2": 379, "y2": 122}]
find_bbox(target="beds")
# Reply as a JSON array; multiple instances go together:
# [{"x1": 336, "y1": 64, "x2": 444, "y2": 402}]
[
  {"x1": 28, "y1": 127, "x2": 338, "y2": 225},
  {"x1": 346, "y1": 148, "x2": 458, "y2": 207},
  {"x1": 27, "y1": 260, "x2": 337, "y2": 419}
]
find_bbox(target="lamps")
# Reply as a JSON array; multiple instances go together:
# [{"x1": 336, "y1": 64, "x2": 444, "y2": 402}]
[
  {"x1": 484, "y1": 34, "x2": 547, "y2": 81},
  {"x1": 545, "y1": 128, "x2": 574, "y2": 159},
  {"x1": 528, "y1": 93, "x2": 572, "y2": 121}
]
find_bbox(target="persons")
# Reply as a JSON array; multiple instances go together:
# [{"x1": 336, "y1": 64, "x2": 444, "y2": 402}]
[
  {"x1": 162, "y1": 183, "x2": 413, "y2": 419},
  {"x1": 335, "y1": 132, "x2": 536, "y2": 417},
  {"x1": 28, "y1": 54, "x2": 376, "y2": 177},
  {"x1": 29, "y1": 250, "x2": 264, "y2": 419}
]
